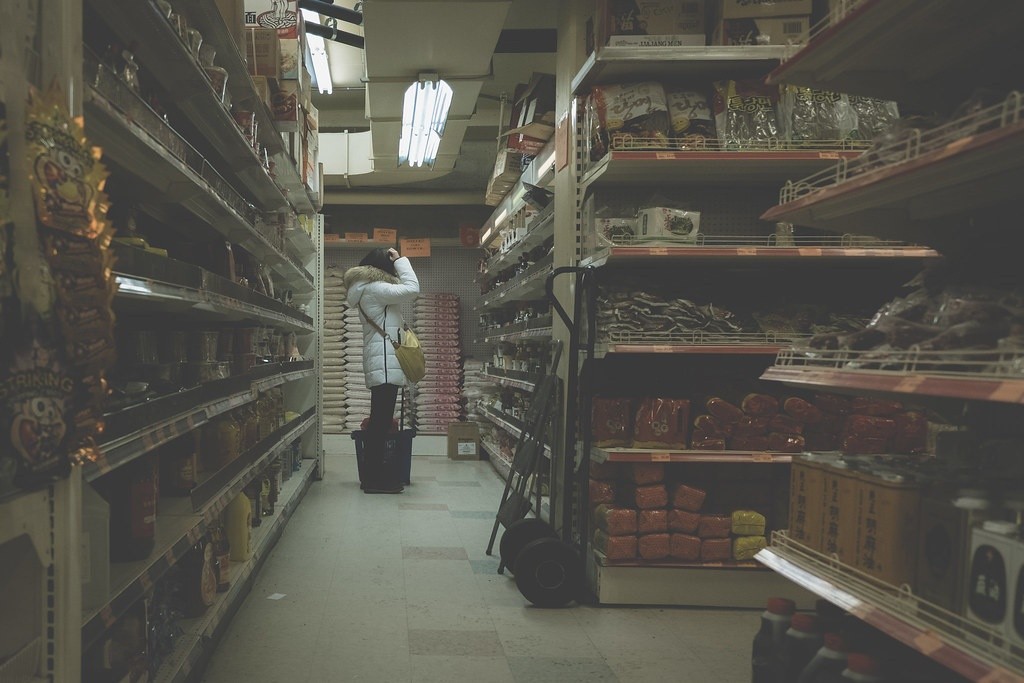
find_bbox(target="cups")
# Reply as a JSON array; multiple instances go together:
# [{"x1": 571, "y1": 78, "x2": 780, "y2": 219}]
[{"x1": 131, "y1": 323, "x2": 284, "y2": 384}]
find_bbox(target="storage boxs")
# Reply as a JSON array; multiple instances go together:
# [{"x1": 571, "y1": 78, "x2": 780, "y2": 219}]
[
  {"x1": 447, "y1": 422, "x2": 481, "y2": 461},
  {"x1": 485, "y1": 72, "x2": 556, "y2": 207},
  {"x1": 594, "y1": 207, "x2": 701, "y2": 244},
  {"x1": 607, "y1": 0, "x2": 815, "y2": 48},
  {"x1": 216, "y1": 0, "x2": 319, "y2": 192},
  {"x1": 791, "y1": 452, "x2": 962, "y2": 609}
]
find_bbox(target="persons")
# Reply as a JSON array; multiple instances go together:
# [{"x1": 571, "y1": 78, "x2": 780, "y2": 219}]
[{"x1": 342, "y1": 247, "x2": 420, "y2": 430}]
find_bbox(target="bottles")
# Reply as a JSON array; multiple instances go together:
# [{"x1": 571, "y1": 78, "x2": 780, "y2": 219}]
[
  {"x1": 282, "y1": 436, "x2": 302, "y2": 480},
  {"x1": 496, "y1": 251, "x2": 529, "y2": 287},
  {"x1": 492, "y1": 339, "x2": 552, "y2": 375},
  {"x1": 751, "y1": 596, "x2": 883, "y2": 683},
  {"x1": 83, "y1": 490, "x2": 251, "y2": 683},
  {"x1": 503, "y1": 390, "x2": 531, "y2": 424},
  {"x1": 125, "y1": 387, "x2": 286, "y2": 539}
]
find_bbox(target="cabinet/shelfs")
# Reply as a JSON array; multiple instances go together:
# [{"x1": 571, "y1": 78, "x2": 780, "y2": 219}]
[
  {"x1": 0, "y1": 0, "x2": 328, "y2": 683},
  {"x1": 753, "y1": 0, "x2": 1024, "y2": 683},
  {"x1": 475, "y1": 0, "x2": 943, "y2": 607}
]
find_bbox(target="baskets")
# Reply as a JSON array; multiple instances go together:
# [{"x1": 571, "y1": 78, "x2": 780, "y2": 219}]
[{"x1": 351, "y1": 428, "x2": 416, "y2": 486}]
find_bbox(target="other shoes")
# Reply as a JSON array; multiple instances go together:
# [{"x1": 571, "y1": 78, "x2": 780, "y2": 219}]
[{"x1": 360, "y1": 476, "x2": 403, "y2": 494}]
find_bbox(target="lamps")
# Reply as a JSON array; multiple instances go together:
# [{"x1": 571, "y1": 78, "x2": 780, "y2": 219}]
[
  {"x1": 397, "y1": 73, "x2": 454, "y2": 170},
  {"x1": 302, "y1": 7, "x2": 334, "y2": 95}
]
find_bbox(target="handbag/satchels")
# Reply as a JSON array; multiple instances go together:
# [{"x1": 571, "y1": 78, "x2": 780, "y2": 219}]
[{"x1": 392, "y1": 324, "x2": 427, "y2": 382}]
